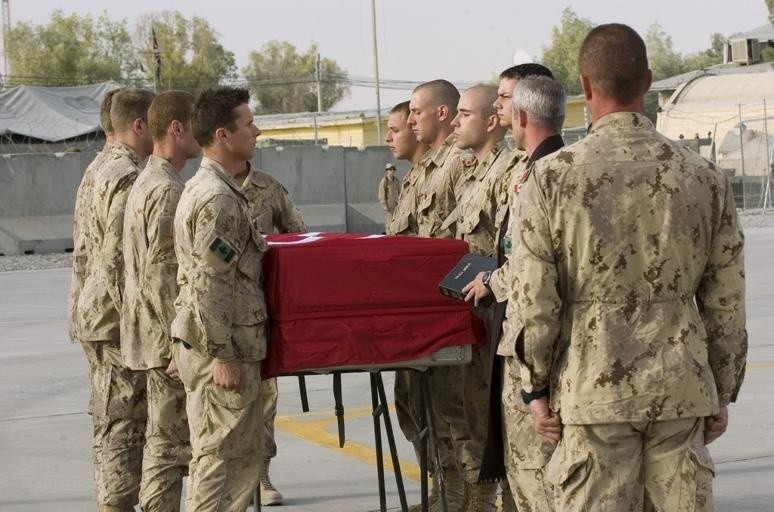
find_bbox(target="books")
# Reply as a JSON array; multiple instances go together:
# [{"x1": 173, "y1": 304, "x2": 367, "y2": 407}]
[{"x1": 439, "y1": 253, "x2": 504, "y2": 308}]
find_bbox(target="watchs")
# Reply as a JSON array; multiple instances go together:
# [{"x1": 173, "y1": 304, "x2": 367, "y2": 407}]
[
  {"x1": 521, "y1": 390, "x2": 550, "y2": 405},
  {"x1": 482, "y1": 271, "x2": 493, "y2": 290}
]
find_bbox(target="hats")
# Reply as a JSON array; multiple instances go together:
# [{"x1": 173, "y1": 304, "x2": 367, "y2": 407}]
[{"x1": 385, "y1": 163, "x2": 395, "y2": 169}]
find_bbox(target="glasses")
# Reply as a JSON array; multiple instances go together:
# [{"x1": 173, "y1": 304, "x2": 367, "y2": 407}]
[{"x1": 388, "y1": 168, "x2": 397, "y2": 171}]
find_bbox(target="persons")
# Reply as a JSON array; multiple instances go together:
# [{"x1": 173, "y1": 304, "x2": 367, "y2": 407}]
[
  {"x1": 406, "y1": 79, "x2": 475, "y2": 512},
  {"x1": 378, "y1": 163, "x2": 400, "y2": 234},
  {"x1": 386, "y1": 101, "x2": 439, "y2": 511},
  {"x1": 490, "y1": 63, "x2": 556, "y2": 257},
  {"x1": 234, "y1": 161, "x2": 309, "y2": 507},
  {"x1": 169, "y1": 88, "x2": 267, "y2": 511},
  {"x1": 120, "y1": 91, "x2": 201, "y2": 512},
  {"x1": 450, "y1": 85, "x2": 512, "y2": 510},
  {"x1": 459, "y1": 76, "x2": 565, "y2": 512},
  {"x1": 68, "y1": 88, "x2": 114, "y2": 506},
  {"x1": 509, "y1": 22, "x2": 751, "y2": 511},
  {"x1": 80, "y1": 90, "x2": 154, "y2": 511}
]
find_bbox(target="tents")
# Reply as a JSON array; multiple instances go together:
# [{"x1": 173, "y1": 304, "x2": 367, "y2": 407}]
[{"x1": 2, "y1": 81, "x2": 133, "y2": 148}]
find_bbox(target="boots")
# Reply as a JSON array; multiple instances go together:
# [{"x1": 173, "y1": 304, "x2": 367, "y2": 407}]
[
  {"x1": 464, "y1": 482, "x2": 497, "y2": 511},
  {"x1": 425, "y1": 473, "x2": 466, "y2": 511},
  {"x1": 408, "y1": 474, "x2": 438, "y2": 511},
  {"x1": 256, "y1": 457, "x2": 284, "y2": 506}
]
